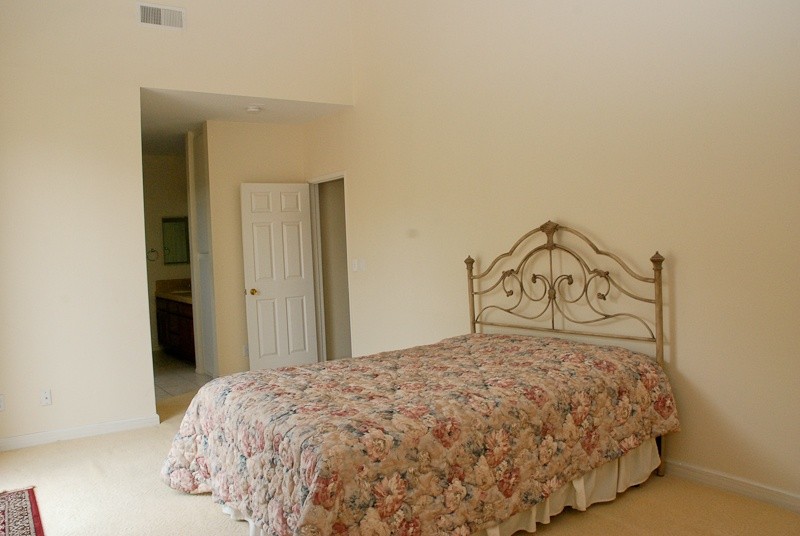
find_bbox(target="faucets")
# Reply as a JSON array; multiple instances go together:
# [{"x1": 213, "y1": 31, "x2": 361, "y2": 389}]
[{"x1": 185, "y1": 281, "x2": 190, "y2": 289}]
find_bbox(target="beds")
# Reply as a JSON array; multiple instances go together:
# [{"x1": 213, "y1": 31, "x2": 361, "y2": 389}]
[{"x1": 161, "y1": 219, "x2": 681, "y2": 536}]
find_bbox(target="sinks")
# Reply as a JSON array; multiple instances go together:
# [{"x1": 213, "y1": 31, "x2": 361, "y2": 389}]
[{"x1": 171, "y1": 290, "x2": 191, "y2": 294}]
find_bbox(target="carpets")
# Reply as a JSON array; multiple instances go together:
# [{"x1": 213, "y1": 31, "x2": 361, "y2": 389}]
[{"x1": 0, "y1": 486, "x2": 45, "y2": 536}]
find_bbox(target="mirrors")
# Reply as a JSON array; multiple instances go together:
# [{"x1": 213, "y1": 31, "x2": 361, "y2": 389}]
[{"x1": 162, "y1": 217, "x2": 190, "y2": 264}]
